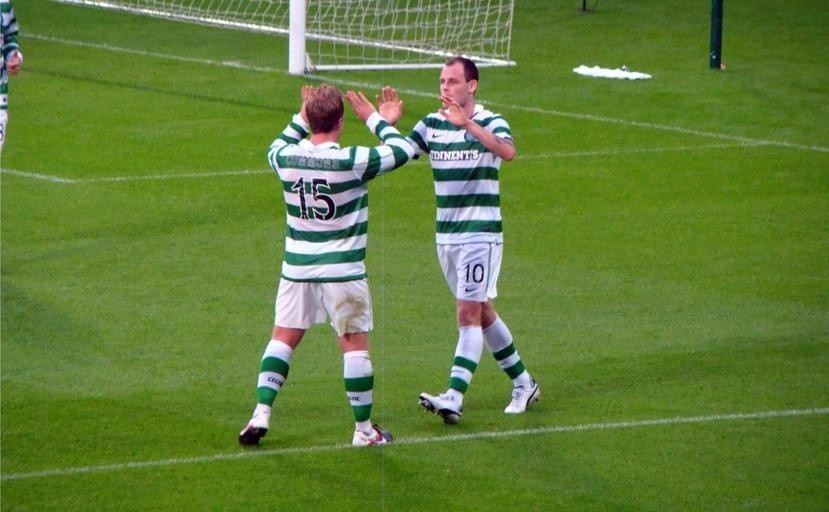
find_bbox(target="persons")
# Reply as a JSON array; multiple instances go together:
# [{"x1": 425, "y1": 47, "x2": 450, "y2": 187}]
[
  {"x1": 0, "y1": 0, "x2": 24, "y2": 153},
  {"x1": 238, "y1": 86, "x2": 394, "y2": 448},
  {"x1": 375, "y1": 56, "x2": 541, "y2": 427}
]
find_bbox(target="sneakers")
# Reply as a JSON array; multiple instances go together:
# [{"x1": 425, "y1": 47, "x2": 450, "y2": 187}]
[
  {"x1": 418, "y1": 392, "x2": 462, "y2": 424},
  {"x1": 505, "y1": 379, "x2": 541, "y2": 414},
  {"x1": 239, "y1": 414, "x2": 269, "y2": 445},
  {"x1": 353, "y1": 428, "x2": 393, "y2": 447}
]
